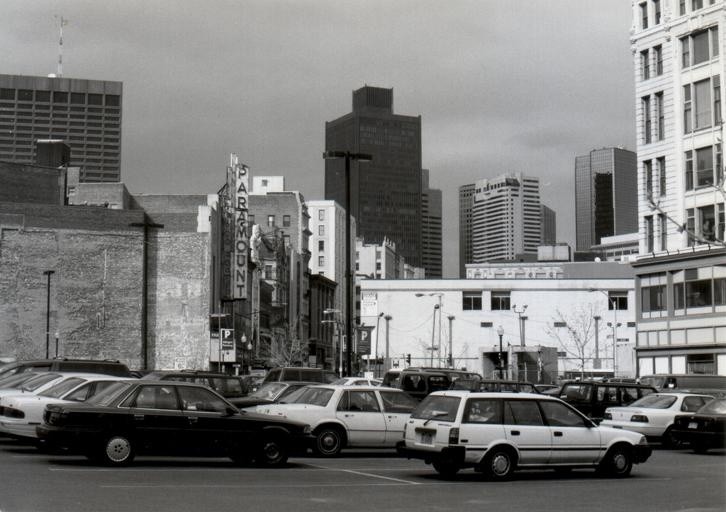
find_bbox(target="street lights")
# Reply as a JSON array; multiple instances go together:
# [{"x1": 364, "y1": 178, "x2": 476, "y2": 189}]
[
  {"x1": 384, "y1": 316, "x2": 392, "y2": 358},
  {"x1": 431, "y1": 304, "x2": 440, "y2": 367},
  {"x1": 448, "y1": 316, "x2": 454, "y2": 366},
  {"x1": 43, "y1": 270, "x2": 55, "y2": 359},
  {"x1": 497, "y1": 325, "x2": 504, "y2": 379},
  {"x1": 241, "y1": 332, "x2": 252, "y2": 376},
  {"x1": 323, "y1": 308, "x2": 343, "y2": 379},
  {"x1": 374, "y1": 312, "x2": 384, "y2": 378},
  {"x1": 55, "y1": 332, "x2": 60, "y2": 359},
  {"x1": 588, "y1": 287, "x2": 617, "y2": 379}
]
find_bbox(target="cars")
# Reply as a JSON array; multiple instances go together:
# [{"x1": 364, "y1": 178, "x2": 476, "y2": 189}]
[
  {"x1": 0, "y1": 370, "x2": 134, "y2": 456},
  {"x1": 402, "y1": 388, "x2": 652, "y2": 479},
  {"x1": 35, "y1": 379, "x2": 314, "y2": 470},
  {"x1": 0, "y1": 359, "x2": 726, "y2": 480},
  {"x1": 223, "y1": 380, "x2": 329, "y2": 409},
  {"x1": 687, "y1": 397, "x2": 726, "y2": 455},
  {"x1": 237, "y1": 383, "x2": 419, "y2": 458},
  {"x1": 601, "y1": 392, "x2": 717, "y2": 450},
  {"x1": 139, "y1": 368, "x2": 273, "y2": 408}
]
